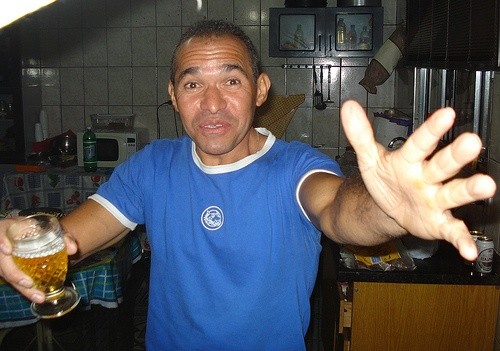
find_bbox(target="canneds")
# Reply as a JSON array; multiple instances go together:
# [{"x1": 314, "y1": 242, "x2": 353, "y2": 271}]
[{"x1": 465, "y1": 231, "x2": 494, "y2": 273}]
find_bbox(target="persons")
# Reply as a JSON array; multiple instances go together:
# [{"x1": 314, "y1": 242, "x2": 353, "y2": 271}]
[{"x1": 0, "y1": 19, "x2": 496, "y2": 351}]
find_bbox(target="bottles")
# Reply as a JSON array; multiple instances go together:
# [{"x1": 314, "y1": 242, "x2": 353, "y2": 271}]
[
  {"x1": 336, "y1": 18, "x2": 347, "y2": 50},
  {"x1": 359, "y1": 26, "x2": 370, "y2": 50},
  {"x1": 83, "y1": 127, "x2": 97, "y2": 173},
  {"x1": 294, "y1": 24, "x2": 304, "y2": 50},
  {"x1": 348, "y1": 24, "x2": 357, "y2": 50}
]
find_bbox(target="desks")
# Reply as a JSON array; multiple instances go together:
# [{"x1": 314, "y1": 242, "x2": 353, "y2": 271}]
[{"x1": 0, "y1": 164, "x2": 143, "y2": 326}]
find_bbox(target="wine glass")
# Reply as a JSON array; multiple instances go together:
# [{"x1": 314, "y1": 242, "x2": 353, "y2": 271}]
[{"x1": 6, "y1": 214, "x2": 82, "y2": 319}]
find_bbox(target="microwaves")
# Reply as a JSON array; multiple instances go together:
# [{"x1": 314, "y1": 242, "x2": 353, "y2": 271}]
[{"x1": 77, "y1": 129, "x2": 136, "y2": 167}]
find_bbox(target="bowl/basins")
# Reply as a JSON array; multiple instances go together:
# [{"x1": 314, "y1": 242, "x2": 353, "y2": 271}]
[{"x1": 90, "y1": 113, "x2": 136, "y2": 131}]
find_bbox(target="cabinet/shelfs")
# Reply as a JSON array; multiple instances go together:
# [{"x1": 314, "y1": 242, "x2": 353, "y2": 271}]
[
  {"x1": 395, "y1": 0, "x2": 500, "y2": 72},
  {"x1": 334, "y1": 282, "x2": 500, "y2": 351},
  {"x1": 268, "y1": 6, "x2": 383, "y2": 58}
]
